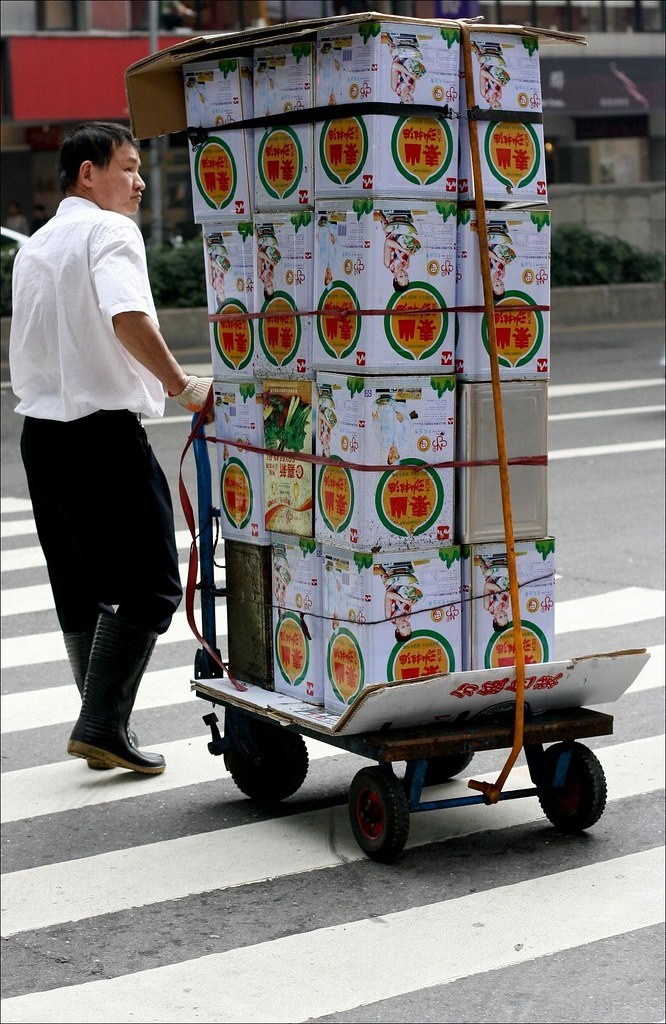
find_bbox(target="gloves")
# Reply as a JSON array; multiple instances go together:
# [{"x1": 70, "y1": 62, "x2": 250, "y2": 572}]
[{"x1": 168, "y1": 375, "x2": 214, "y2": 426}]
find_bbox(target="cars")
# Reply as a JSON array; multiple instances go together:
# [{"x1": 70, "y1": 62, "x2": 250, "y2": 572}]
[{"x1": 0, "y1": 227, "x2": 30, "y2": 254}]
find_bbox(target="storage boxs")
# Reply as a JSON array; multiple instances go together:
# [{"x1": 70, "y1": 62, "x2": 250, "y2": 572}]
[{"x1": 180, "y1": 5, "x2": 558, "y2": 718}]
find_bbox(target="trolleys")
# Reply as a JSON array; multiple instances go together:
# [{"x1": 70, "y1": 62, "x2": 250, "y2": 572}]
[{"x1": 189, "y1": 408, "x2": 614, "y2": 865}]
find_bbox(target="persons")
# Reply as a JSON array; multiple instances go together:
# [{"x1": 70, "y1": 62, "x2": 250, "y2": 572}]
[{"x1": 8, "y1": 121, "x2": 214, "y2": 775}]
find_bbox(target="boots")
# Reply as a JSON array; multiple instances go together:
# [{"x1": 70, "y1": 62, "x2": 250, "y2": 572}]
[{"x1": 60, "y1": 613, "x2": 165, "y2": 774}]
